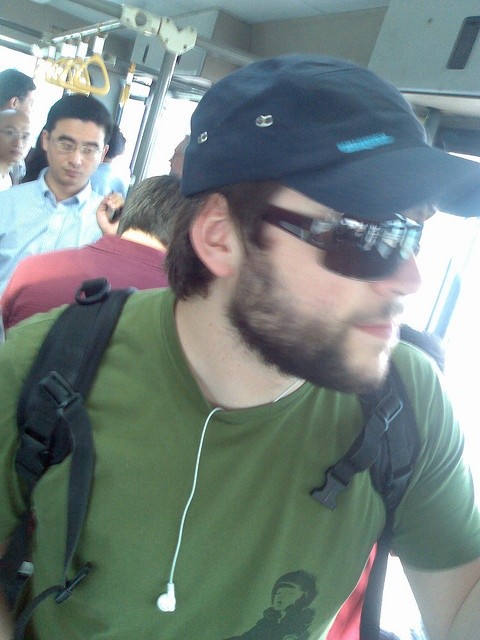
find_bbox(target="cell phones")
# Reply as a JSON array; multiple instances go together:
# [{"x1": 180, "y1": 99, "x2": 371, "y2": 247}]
[{"x1": 107, "y1": 190, "x2": 122, "y2": 224}]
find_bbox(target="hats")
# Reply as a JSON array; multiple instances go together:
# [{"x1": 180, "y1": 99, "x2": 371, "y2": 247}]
[{"x1": 182, "y1": 53, "x2": 480, "y2": 223}]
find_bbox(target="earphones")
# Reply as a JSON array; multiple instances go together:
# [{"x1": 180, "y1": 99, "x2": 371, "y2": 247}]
[{"x1": 156, "y1": 583, "x2": 177, "y2": 616}]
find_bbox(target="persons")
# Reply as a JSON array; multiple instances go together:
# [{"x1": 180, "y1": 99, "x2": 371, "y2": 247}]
[
  {"x1": 325, "y1": 316, "x2": 445, "y2": 638},
  {"x1": 90, "y1": 122, "x2": 131, "y2": 199},
  {"x1": 0, "y1": 107, "x2": 32, "y2": 190},
  {"x1": 0, "y1": 49, "x2": 480, "y2": 640},
  {"x1": 0, "y1": 175, "x2": 184, "y2": 328},
  {"x1": 227, "y1": 568, "x2": 319, "y2": 639},
  {"x1": 167, "y1": 133, "x2": 192, "y2": 179},
  {"x1": 0, "y1": 93, "x2": 114, "y2": 294},
  {"x1": 0, "y1": 68, "x2": 37, "y2": 115}
]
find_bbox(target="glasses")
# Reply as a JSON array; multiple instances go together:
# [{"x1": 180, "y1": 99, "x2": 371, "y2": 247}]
[
  {"x1": 1, "y1": 130, "x2": 32, "y2": 142},
  {"x1": 259, "y1": 203, "x2": 422, "y2": 279},
  {"x1": 50, "y1": 133, "x2": 105, "y2": 158}
]
